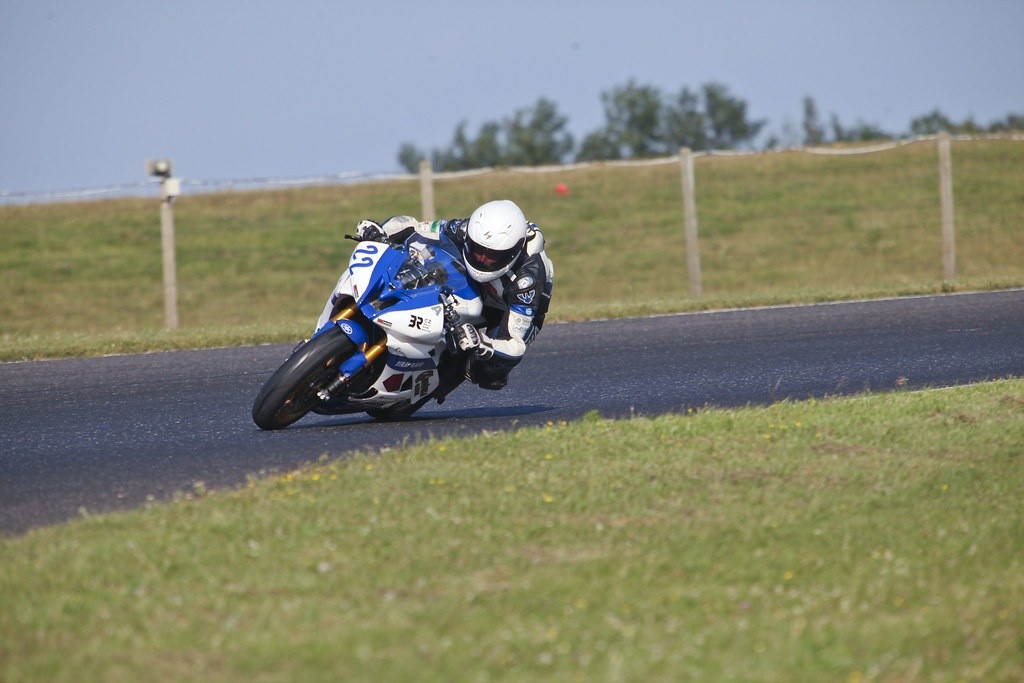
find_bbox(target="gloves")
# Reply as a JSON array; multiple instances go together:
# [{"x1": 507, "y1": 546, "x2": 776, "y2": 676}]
[
  {"x1": 451, "y1": 323, "x2": 494, "y2": 362},
  {"x1": 356, "y1": 220, "x2": 385, "y2": 244}
]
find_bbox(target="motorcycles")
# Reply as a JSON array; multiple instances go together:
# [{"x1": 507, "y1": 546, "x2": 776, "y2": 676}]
[{"x1": 250, "y1": 210, "x2": 528, "y2": 431}]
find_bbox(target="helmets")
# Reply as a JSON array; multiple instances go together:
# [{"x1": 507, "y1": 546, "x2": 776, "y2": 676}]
[{"x1": 464, "y1": 199, "x2": 527, "y2": 283}]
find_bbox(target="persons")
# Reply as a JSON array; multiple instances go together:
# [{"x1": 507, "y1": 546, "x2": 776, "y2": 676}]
[{"x1": 355, "y1": 200, "x2": 554, "y2": 387}]
